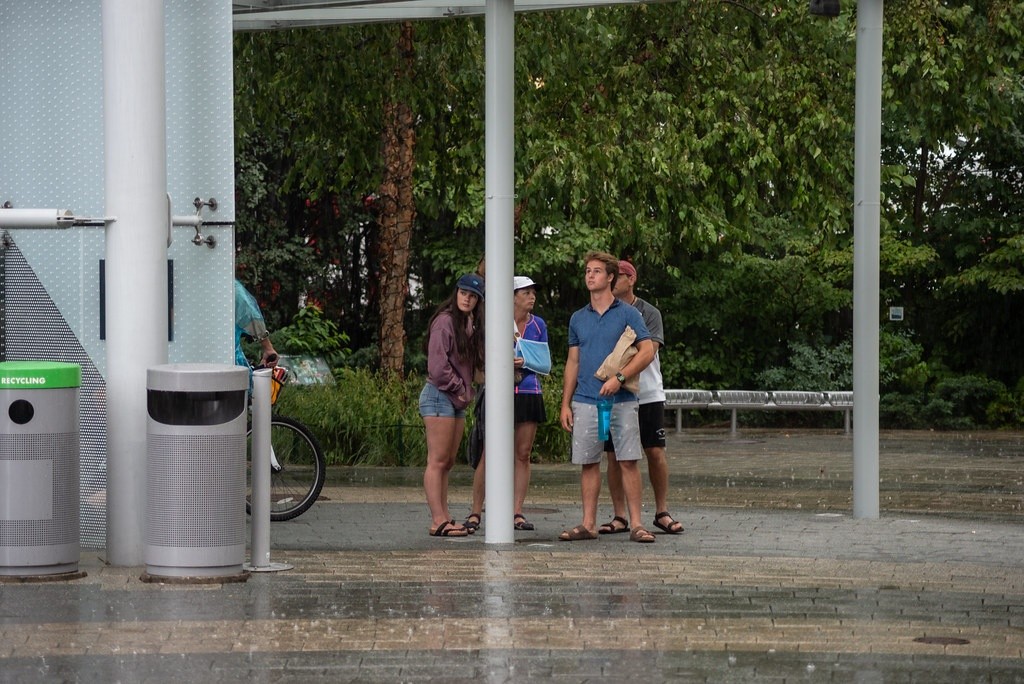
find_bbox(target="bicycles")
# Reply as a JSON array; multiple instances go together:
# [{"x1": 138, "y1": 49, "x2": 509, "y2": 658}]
[{"x1": 246, "y1": 354, "x2": 327, "y2": 522}]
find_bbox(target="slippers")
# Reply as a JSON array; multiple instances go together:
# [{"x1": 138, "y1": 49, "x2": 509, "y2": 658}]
[
  {"x1": 559, "y1": 525, "x2": 596, "y2": 539},
  {"x1": 631, "y1": 526, "x2": 655, "y2": 542},
  {"x1": 429, "y1": 519, "x2": 475, "y2": 537}
]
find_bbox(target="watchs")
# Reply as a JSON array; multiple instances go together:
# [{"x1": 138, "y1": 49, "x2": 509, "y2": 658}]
[{"x1": 615, "y1": 373, "x2": 626, "y2": 386}]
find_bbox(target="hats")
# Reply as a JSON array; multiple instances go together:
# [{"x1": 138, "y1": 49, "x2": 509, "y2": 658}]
[
  {"x1": 456, "y1": 273, "x2": 484, "y2": 300},
  {"x1": 514, "y1": 275, "x2": 535, "y2": 289},
  {"x1": 618, "y1": 261, "x2": 636, "y2": 276}
]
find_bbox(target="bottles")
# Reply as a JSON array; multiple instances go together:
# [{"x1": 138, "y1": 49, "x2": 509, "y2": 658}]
[{"x1": 596, "y1": 399, "x2": 612, "y2": 441}]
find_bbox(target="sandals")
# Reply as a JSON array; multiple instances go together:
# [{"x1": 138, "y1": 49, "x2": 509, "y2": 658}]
[
  {"x1": 514, "y1": 514, "x2": 534, "y2": 530},
  {"x1": 652, "y1": 510, "x2": 685, "y2": 534},
  {"x1": 466, "y1": 513, "x2": 481, "y2": 530},
  {"x1": 600, "y1": 516, "x2": 629, "y2": 533}
]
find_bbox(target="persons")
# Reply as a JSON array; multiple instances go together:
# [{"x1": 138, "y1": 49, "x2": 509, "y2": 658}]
[
  {"x1": 560, "y1": 251, "x2": 657, "y2": 543},
  {"x1": 234, "y1": 276, "x2": 280, "y2": 392},
  {"x1": 597, "y1": 261, "x2": 684, "y2": 534},
  {"x1": 464, "y1": 276, "x2": 552, "y2": 530},
  {"x1": 417, "y1": 274, "x2": 486, "y2": 536}
]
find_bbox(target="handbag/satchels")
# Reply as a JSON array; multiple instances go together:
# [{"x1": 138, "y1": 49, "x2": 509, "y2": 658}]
[
  {"x1": 596, "y1": 327, "x2": 642, "y2": 393},
  {"x1": 272, "y1": 377, "x2": 284, "y2": 406}
]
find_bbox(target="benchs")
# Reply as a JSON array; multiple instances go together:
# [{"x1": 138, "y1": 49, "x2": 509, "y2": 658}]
[{"x1": 665, "y1": 390, "x2": 854, "y2": 434}]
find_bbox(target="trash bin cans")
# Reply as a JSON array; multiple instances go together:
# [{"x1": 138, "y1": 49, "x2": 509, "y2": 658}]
[
  {"x1": 0, "y1": 363, "x2": 81, "y2": 575},
  {"x1": 144, "y1": 365, "x2": 250, "y2": 577}
]
